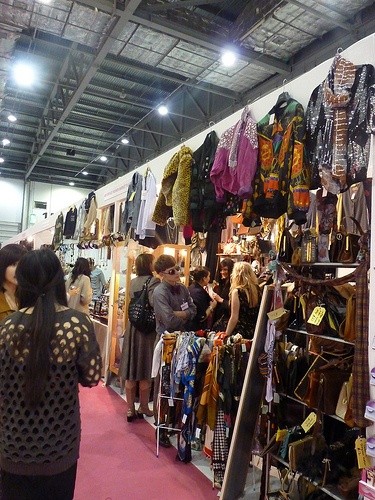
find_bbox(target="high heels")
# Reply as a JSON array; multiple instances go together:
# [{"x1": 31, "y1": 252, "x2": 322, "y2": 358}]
[
  {"x1": 137, "y1": 406, "x2": 154, "y2": 419},
  {"x1": 127, "y1": 410, "x2": 138, "y2": 422}
]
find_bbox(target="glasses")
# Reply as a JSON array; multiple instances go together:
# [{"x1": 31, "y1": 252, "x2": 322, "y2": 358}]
[{"x1": 163, "y1": 266, "x2": 180, "y2": 275}]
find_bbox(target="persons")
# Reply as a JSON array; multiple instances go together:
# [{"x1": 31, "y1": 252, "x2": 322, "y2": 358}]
[
  {"x1": 224, "y1": 261, "x2": 263, "y2": 340},
  {"x1": 65, "y1": 257, "x2": 93, "y2": 316},
  {"x1": 153, "y1": 254, "x2": 197, "y2": 447},
  {"x1": 118, "y1": 252, "x2": 162, "y2": 421},
  {"x1": 87, "y1": 258, "x2": 106, "y2": 300},
  {"x1": 214, "y1": 258, "x2": 236, "y2": 325},
  {"x1": 188, "y1": 266, "x2": 218, "y2": 330},
  {"x1": 0, "y1": 244, "x2": 30, "y2": 320},
  {"x1": 0, "y1": 247, "x2": 102, "y2": 500}
]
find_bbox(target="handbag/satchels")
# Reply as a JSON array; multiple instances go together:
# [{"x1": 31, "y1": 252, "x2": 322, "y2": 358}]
[
  {"x1": 128, "y1": 276, "x2": 156, "y2": 334},
  {"x1": 260, "y1": 277, "x2": 363, "y2": 500},
  {"x1": 278, "y1": 216, "x2": 364, "y2": 264}
]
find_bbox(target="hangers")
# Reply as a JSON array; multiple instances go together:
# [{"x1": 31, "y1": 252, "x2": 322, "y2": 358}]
[
  {"x1": 241, "y1": 100, "x2": 250, "y2": 116},
  {"x1": 207, "y1": 121, "x2": 216, "y2": 139},
  {"x1": 135, "y1": 166, "x2": 138, "y2": 172},
  {"x1": 334, "y1": 48, "x2": 344, "y2": 63},
  {"x1": 146, "y1": 160, "x2": 151, "y2": 174},
  {"x1": 276, "y1": 79, "x2": 290, "y2": 106},
  {"x1": 180, "y1": 136, "x2": 186, "y2": 147}
]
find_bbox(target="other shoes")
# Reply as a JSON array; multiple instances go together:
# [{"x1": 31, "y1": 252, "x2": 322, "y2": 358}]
[{"x1": 156, "y1": 423, "x2": 178, "y2": 449}]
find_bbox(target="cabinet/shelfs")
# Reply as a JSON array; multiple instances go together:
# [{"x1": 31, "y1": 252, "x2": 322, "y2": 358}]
[{"x1": 263, "y1": 255, "x2": 368, "y2": 500}]
